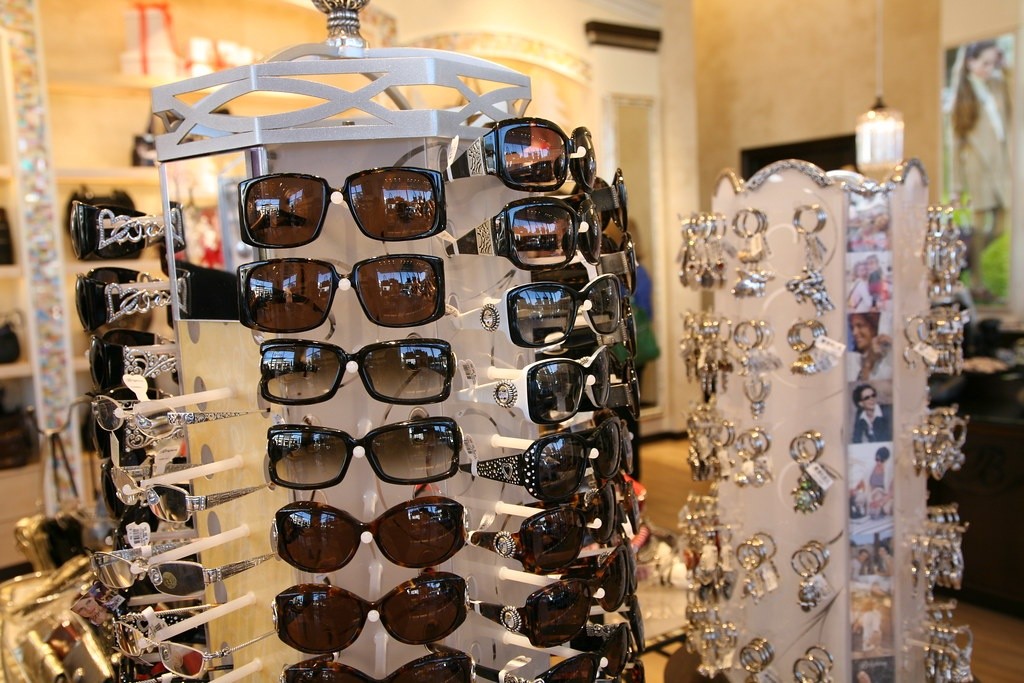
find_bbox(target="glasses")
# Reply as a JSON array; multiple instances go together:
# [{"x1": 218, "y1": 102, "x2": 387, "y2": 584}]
[
  {"x1": 874, "y1": 457, "x2": 884, "y2": 463},
  {"x1": 859, "y1": 393, "x2": 876, "y2": 403},
  {"x1": 68, "y1": 116, "x2": 647, "y2": 683}
]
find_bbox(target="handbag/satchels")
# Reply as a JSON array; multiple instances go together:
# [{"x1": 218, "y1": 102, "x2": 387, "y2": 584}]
[
  {"x1": 68, "y1": 182, "x2": 142, "y2": 256},
  {"x1": 17, "y1": 428, "x2": 87, "y2": 571}
]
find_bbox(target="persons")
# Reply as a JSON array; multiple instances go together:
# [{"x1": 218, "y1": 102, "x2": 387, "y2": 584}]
[
  {"x1": 845, "y1": 213, "x2": 895, "y2": 311},
  {"x1": 852, "y1": 447, "x2": 896, "y2": 507},
  {"x1": 71, "y1": 594, "x2": 113, "y2": 627},
  {"x1": 602, "y1": 220, "x2": 659, "y2": 527},
  {"x1": 850, "y1": 544, "x2": 893, "y2": 683},
  {"x1": 846, "y1": 312, "x2": 892, "y2": 380},
  {"x1": 852, "y1": 385, "x2": 894, "y2": 443}
]
